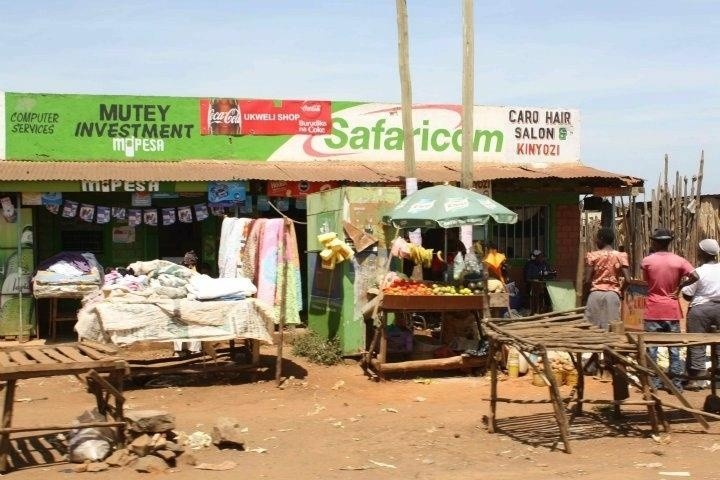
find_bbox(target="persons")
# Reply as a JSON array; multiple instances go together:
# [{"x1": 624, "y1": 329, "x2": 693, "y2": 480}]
[
  {"x1": 584, "y1": 228, "x2": 631, "y2": 375},
  {"x1": 524, "y1": 250, "x2": 554, "y2": 281},
  {"x1": 639, "y1": 229, "x2": 700, "y2": 395},
  {"x1": 682, "y1": 238, "x2": 720, "y2": 390}
]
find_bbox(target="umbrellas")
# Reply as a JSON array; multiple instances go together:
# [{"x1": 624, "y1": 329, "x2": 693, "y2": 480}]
[{"x1": 382, "y1": 181, "x2": 519, "y2": 262}]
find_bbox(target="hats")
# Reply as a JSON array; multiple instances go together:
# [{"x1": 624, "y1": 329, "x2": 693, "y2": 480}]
[
  {"x1": 701, "y1": 239, "x2": 719, "y2": 255},
  {"x1": 650, "y1": 228, "x2": 673, "y2": 241}
]
